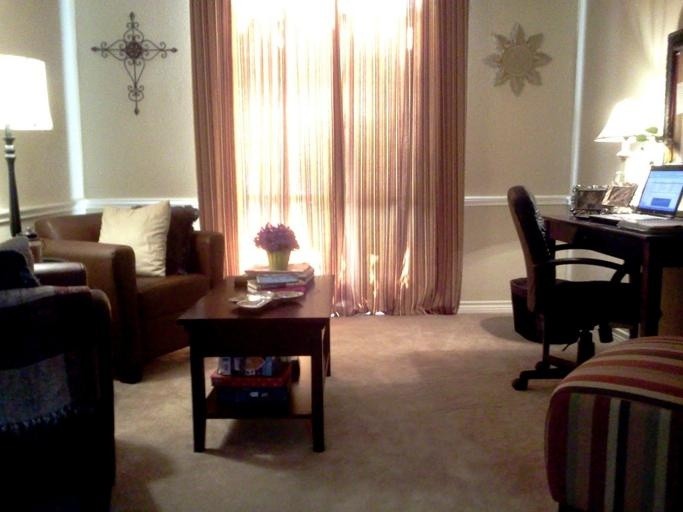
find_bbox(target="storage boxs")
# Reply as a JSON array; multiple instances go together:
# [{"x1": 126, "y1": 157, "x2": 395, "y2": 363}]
[{"x1": 210, "y1": 361, "x2": 291, "y2": 402}]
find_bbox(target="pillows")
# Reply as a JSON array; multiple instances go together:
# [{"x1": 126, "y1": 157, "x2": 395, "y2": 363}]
[
  {"x1": 97, "y1": 198, "x2": 200, "y2": 279},
  {"x1": 0, "y1": 235, "x2": 41, "y2": 292}
]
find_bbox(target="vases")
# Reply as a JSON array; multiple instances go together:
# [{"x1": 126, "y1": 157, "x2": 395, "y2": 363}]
[{"x1": 266, "y1": 249, "x2": 290, "y2": 271}]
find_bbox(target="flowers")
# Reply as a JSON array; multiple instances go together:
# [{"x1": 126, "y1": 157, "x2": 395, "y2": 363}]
[{"x1": 252, "y1": 221, "x2": 301, "y2": 254}]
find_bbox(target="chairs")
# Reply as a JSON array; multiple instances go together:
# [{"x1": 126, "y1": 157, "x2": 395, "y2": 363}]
[
  {"x1": 32, "y1": 212, "x2": 226, "y2": 383},
  {"x1": 0, "y1": 262, "x2": 116, "y2": 511},
  {"x1": 506, "y1": 184, "x2": 664, "y2": 390}
]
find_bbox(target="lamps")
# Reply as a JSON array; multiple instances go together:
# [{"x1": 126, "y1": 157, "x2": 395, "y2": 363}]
[{"x1": 0, "y1": 53, "x2": 53, "y2": 240}]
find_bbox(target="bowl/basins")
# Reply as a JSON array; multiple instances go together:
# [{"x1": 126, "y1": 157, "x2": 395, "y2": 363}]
[{"x1": 573, "y1": 183, "x2": 606, "y2": 205}]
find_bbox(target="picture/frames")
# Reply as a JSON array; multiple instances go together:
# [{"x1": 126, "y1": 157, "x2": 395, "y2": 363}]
[{"x1": 568, "y1": 181, "x2": 638, "y2": 220}]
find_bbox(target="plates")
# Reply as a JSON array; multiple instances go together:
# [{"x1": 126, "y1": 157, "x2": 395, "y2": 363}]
[{"x1": 228, "y1": 292, "x2": 305, "y2": 303}]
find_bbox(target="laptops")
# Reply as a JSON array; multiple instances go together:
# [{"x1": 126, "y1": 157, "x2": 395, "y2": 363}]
[{"x1": 589, "y1": 165, "x2": 683, "y2": 224}]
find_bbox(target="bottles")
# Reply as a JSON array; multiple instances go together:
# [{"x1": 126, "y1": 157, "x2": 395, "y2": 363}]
[{"x1": 20, "y1": 231, "x2": 44, "y2": 263}]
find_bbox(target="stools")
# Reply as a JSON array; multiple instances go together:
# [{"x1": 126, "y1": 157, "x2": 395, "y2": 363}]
[{"x1": 541, "y1": 337, "x2": 682, "y2": 510}]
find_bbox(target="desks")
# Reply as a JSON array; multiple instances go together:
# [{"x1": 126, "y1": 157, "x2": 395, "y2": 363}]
[{"x1": 540, "y1": 209, "x2": 682, "y2": 336}]
